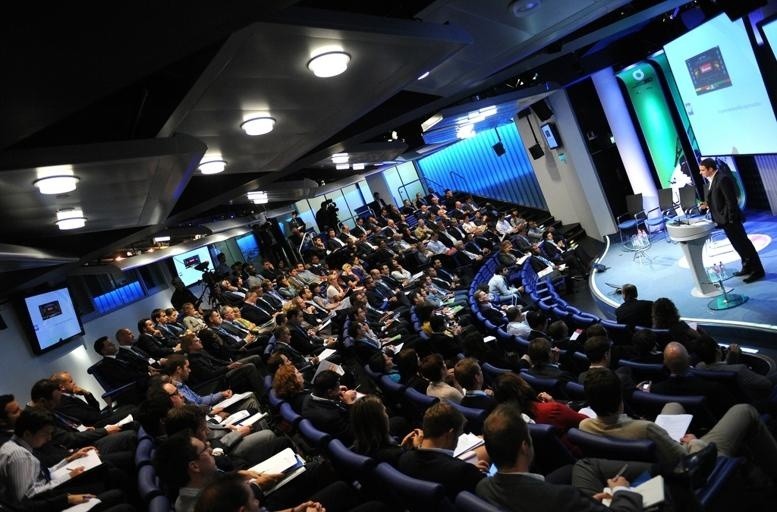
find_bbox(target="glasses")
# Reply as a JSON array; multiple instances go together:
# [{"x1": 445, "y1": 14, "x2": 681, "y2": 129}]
[
  {"x1": 200, "y1": 441, "x2": 207, "y2": 455},
  {"x1": 169, "y1": 389, "x2": 178, "y2": 398}
]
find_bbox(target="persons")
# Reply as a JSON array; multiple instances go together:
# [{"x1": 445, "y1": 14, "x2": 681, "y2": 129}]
[{"x1": 2, "y1": 157, "x2": 777, "y2": 512}]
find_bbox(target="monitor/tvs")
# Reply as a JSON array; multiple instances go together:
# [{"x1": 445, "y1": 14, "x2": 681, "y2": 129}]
[
  {"x1": 540, "y1": 122, "x2": 560, "y2": 149},
  {"x1": 170, "y1": 246, "x2": 215, "y2": 288},
  {"x1": 23, "y1": 287, "x2": 84, "y2": 355}
]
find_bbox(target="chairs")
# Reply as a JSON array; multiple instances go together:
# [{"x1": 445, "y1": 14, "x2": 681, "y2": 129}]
[
  {"x1": 616, "y1": 192, "x2": 648, "y2": 244},
  {"x1": 646, "y1": 188, "x2": 678, "y2": 242},
  {"x1": 678, "y1": 183, "x2": 700, "y2": 218},
  {"x1": 339, "y1": 199, "x2": 605, "y2": 324}
]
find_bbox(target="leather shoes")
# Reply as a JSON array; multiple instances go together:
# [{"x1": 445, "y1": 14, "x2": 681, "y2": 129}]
[{"x1": 733, "y1": 268, "x2": 751, "y2": 276}]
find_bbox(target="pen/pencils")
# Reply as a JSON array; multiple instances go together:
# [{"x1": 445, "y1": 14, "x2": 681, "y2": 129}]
[
  {"x1": 258, "y1": 471, "x2": 265, "y2": 476},
  {"x1": 238, "y1": 422, "x2": 243, "y2": 426},
  {"x1": 353, "y1": 384, "x2": 362, "y2": 391},
  {"x1": 66, "y1": 468, "x2": 73, "y2": 470},
  {"x1": 612, "y1": 464, "x2": 628, "y2": 482}
]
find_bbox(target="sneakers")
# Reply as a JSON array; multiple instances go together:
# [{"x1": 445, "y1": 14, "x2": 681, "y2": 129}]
[{"x1": 744, "y1": 272, "x2": 766, "y2": 283}]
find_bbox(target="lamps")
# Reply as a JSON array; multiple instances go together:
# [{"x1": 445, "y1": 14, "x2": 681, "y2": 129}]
[
  {"x1": 31, "y1": 175, "x2": 79, "y2": 196},
  {"x1": 305, "y1": 48, "x2": 354, "y2": 79},
  {"x1": 197, "y1": 156, "x2": 227, "y2": 176},
  {"x1": 238, "y1": 113, "x2": 276, "y2": 137},
  {"x1": 49, "y1": 210, "x2": 90, "y2": 232}
]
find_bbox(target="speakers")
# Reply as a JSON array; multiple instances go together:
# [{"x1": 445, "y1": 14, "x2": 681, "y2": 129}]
[
  {"x1": 529, "y1": 144, "x2": 544, "y2": 160},
  {"x1": 493, "y1": 142, "x2": 505, "y2": 157}
]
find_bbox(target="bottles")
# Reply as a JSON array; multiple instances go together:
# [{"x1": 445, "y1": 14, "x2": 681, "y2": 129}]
[
  {"x1": 637, "y1": 229, "x2": 648, "y2": 245},
  {"x1": 713, "y1": 260, "x2": 726, "y2": 279}
]
